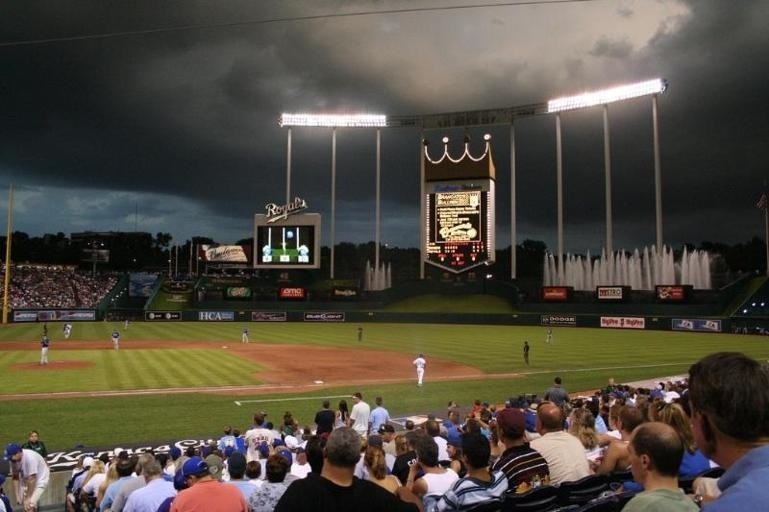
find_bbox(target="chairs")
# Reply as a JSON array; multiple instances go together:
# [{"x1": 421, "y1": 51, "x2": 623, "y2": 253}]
[
  {"x1": 448, "y1": 468, "x2": 634, "y2": 512},
  {"x1": 677, "y1": 463, "x2": 728, "y2": 496}
]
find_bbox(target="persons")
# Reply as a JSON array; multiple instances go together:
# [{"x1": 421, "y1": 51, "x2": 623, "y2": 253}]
[
  {"x1": 356, "y1": 325, "x2": 364, "y2": 340},
  {"x1": 111, "y1": 328, "x2": 120, "y2": 352},
  {"x1": 2, "y1": 377, "x2": 722, "y2": 512},
  {"x1": 412, "y1": 354, "x2": 426, "y2": 385},
  {"x1": 523, "y1": 341, "x2": 531, "y2": 365},
  {"x1": 242, "y1": 328, "x2": 249, "y2": 343},
  {"x1": 42, "y1": 322, "x2": 49, "y2": 334},
  {"x1": 689, "y1": 352, "x2": 769, "y2": 512},
  {"x1": 0, "y1": 264, "x2": 120, "y2": 307},
  {"x1": 64, "y1": 322, "x2": 72, "y2": 338},
  {"x1": 545, "y1": 327, "x2": 553, "y2": 344},
  {"x1": 39, "y1": 334, "x2": 55, "y2": 364}
]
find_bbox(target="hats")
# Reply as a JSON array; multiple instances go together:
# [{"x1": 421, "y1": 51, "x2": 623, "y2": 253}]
[
  {"x1": 4, "y1": 443, "x2": 19, "y2": 460},
  {"x1": 527, "y1": 402, "x2": 538, "y2": 413}
]
